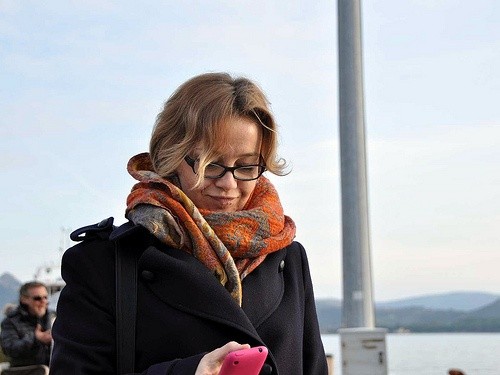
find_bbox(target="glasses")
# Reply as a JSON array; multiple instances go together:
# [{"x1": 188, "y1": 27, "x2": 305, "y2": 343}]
[
  {"x1": 180, "y1": 151, "x2": 268, "y2": 182},
  {"x1": 25, "y1": 294, "x2": 48, "y2": 301}
]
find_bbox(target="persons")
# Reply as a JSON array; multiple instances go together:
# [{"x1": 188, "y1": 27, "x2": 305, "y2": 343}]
[
  {"x1": 49, "y1": 71, "x2": 329, "y2": 375},
  {"x1": 0, "y1": 281, "x2": 56, "y2": 375}
]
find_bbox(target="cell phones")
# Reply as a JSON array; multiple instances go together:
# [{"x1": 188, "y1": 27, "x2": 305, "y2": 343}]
[{"x1": 217, "y1": 346, "x2": 268, "y2": 374}]
